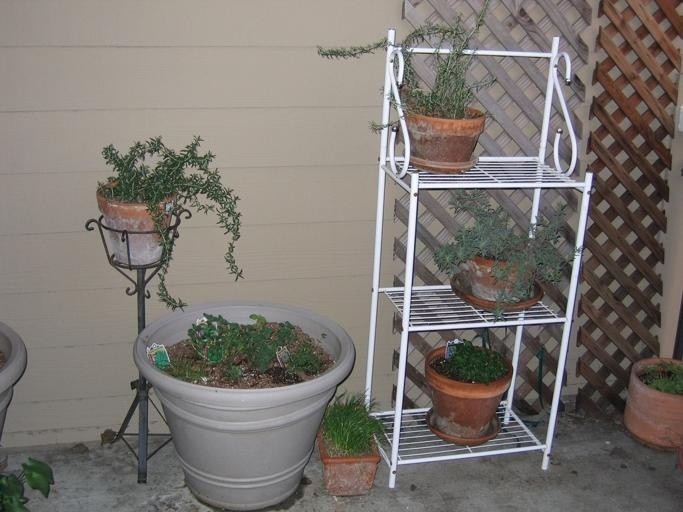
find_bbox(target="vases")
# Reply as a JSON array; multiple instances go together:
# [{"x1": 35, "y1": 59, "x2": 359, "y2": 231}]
[{"x1": 1, "y1": 321, "x2": 28, "y2": 471}]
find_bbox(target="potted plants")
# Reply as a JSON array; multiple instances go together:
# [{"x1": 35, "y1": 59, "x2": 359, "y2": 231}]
[
  {"x1": 316, "y1": 0, "x2": 500, "y2": 175},
  {"x1": 133, "y1": 303, "x2": 355, "y2": 512},
  {"x1": 96, "y1": 136, "x2": 243, "y2": 310},
  {"x1": 317, "y1": 389, "x2": 384, "y2": 497},
  {"x1": 433, "y1": 187, "x2": 583, "y2": 325},
  {"x1": 424, "y1": 333, "x2": 513, "y2": 446},
  {"x1": 623, "y1": 358, "x2": 683, "y2": 452}
]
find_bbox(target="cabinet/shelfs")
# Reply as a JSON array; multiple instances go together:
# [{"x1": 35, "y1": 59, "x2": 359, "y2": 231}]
[{"x1": 362, "y1": 30, "x2": 594, "y2": 490}]
